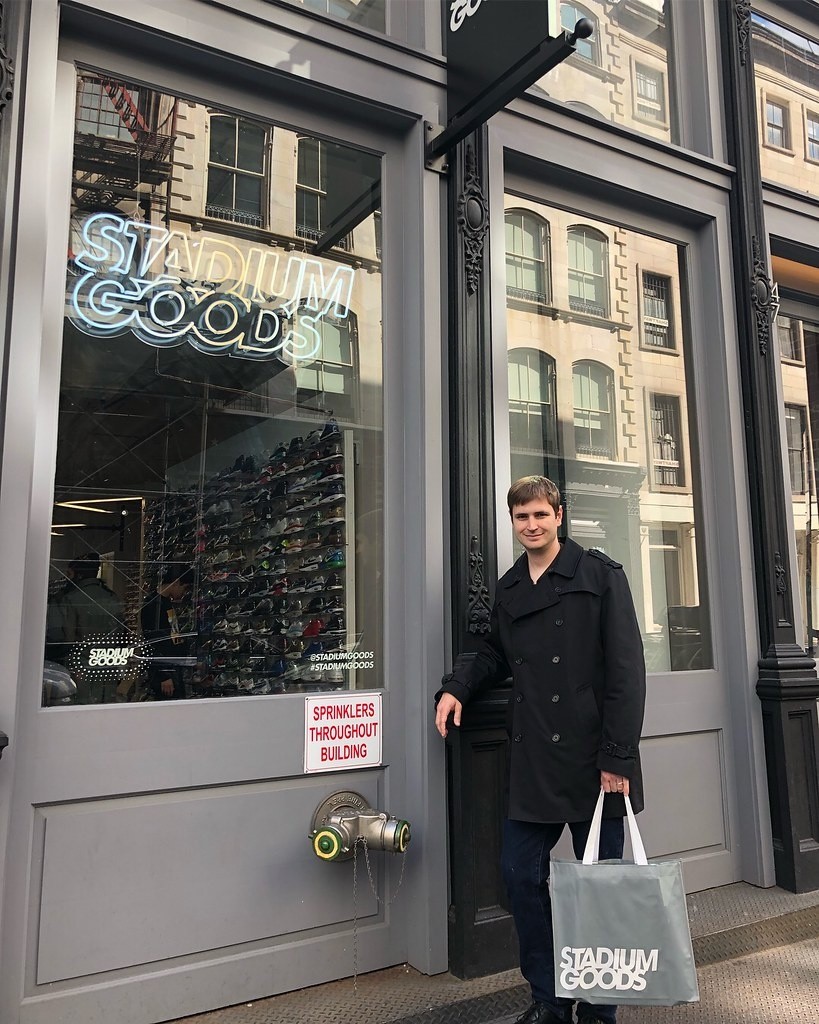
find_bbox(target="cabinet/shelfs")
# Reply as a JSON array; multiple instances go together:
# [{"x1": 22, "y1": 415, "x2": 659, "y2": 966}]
[{"x1": 147, "y1": 429, "x2": 356, "y2": 699}]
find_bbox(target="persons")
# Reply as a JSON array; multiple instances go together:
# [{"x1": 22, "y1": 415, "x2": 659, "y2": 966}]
[
  {"x1": 46, "y1": 551, "x2": 127, "y2": 706},
  {"x1": 434, "y1": 476, "x2": 648, "y2": 1024},
  {"x1": 140, "y1": 563, "x2": 196, "y2": 701}
]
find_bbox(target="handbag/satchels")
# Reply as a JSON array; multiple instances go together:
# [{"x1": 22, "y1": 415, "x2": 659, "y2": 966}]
[{"x1": 549, "y1": 784, "x2": 700, "y2": 1007}]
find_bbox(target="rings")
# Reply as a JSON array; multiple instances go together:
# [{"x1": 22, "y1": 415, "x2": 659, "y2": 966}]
[{"x1": 617, "y1": 782, "x2": 623, "y2": 785}]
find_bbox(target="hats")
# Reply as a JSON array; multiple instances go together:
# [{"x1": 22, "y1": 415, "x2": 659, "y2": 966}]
[{"x1": 68, "y1": 552, "x2": 101, "y2": 579}]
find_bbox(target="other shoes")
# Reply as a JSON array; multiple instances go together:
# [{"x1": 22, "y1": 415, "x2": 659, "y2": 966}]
[
  {"x1": 513, "y1": 1000, "x2": 574, "y2": 1024},
  {"x1": 577, "y1": 1002, "x2": 610, "y2": 1024},
  {"x1": 124, "y1": 417, "x2": 346, "y2": 698}
]
